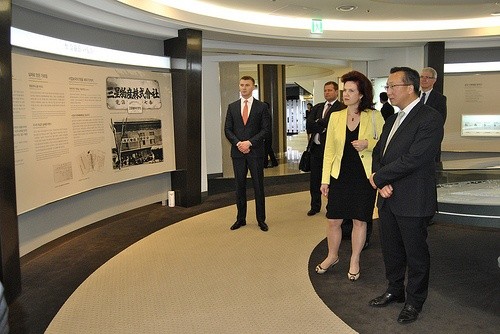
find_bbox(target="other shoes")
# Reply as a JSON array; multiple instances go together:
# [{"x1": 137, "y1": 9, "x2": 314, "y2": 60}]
[
  {"x1": 363, "y1": 232, "x2": 372, "y2": 251},
  {"x1": 307, "y1": 209, "x2": 320, "y2": 216},
  {"x1": 264, "y1": 163, "x2": 268, "y2": 168},
  {"x1": 269, "y1": 162, "x2": 278, "y2": 168},
  {"x1": 342, "y1": 231, "x2": 351, "y2": 240},
  {"x1": 427, "y1": 218, "x2": 434, "y2": 226}
]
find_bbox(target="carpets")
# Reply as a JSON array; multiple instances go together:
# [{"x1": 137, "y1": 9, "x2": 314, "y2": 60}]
[{"x1": 307, "y1": 216, "x2": 500, "y2": 334}]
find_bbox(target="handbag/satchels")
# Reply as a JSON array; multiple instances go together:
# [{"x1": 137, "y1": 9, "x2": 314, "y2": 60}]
[{"x1": 299, "y1": 151, "x2": 312, "y2": 172}]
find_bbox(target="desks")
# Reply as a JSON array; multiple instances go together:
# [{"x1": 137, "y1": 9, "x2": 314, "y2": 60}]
[{"x1": 431, "y1": 179, "x2": 500, "y2": 270}]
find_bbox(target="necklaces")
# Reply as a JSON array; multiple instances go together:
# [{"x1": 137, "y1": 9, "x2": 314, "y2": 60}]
[{"x1": 347, "y1": 108, "x2": 360, "y2": 121}]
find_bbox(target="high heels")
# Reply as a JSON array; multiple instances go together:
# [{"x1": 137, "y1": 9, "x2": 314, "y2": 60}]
[
  {"x1": 348, "y1": 258, "x2": 361, "y2": 281},
  {"x1": 315, "y1": 257, "x2": 340, "y2": 274}
]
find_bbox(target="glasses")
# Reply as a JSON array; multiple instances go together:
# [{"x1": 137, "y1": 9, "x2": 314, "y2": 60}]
[
  {"x1": 384, "y1": 82, "x2": 415, "y2": 91},
  {"x1": 420, "y1": 75, "x2": 434, "y2": 80}
]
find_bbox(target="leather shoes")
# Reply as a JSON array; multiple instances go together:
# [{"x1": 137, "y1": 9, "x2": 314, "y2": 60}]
[
  {"x1": 230, "y1": 219, "x2": 246, "y2": 230},
  {"x1": 369, "y1": 292, "x2": 406, "y2": 307},
  {"x1": 398, "y1": 302, "x2": 422, "y2": 324},
  {"x1": 258, "y1": 221, "x2": 268, "y2": 231}
]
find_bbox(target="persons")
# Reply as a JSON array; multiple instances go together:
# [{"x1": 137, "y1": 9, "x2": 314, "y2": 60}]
[
  {"x1": 120, "y1": 151, "x2": 154, "y2": 167},
  {"x1": 367, "y1": 67, "x2": 444, "y2": 323},
  {"x1": 306, "y1": 81, "x2": 348, "y2": 219},
  {"x1": 304, "y1": 102, "x2": 314, "y2": 143},
  {"x1": 416, "y1": 68, "x2": 448, "y2": 130},
  {"x1": 260, "y1": 101, "x2": 279, "y2": 169},
  {"x1": 224, "y1": 76, "x2": 271, "y2": 231},
  {"x1": 378, "y1": 92, "x2": 394, "y2": 122},
  {"x1": 314, "y1": 71, "x2": 388, "y2": 280}
]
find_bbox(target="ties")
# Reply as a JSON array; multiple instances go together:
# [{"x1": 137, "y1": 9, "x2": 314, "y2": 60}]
[
  {"x1": 324, "y1": 103, "x2": 332, "y2": 118},
  {"x1": 420, "y1": 93, "x2": 426, "y2": 104},
  {"x1": 242, "y1": 100, "x2": 249, "y2": 125},
  {"x1": 382, "y1": 112, "x2": 405, "y2": 157}
]
